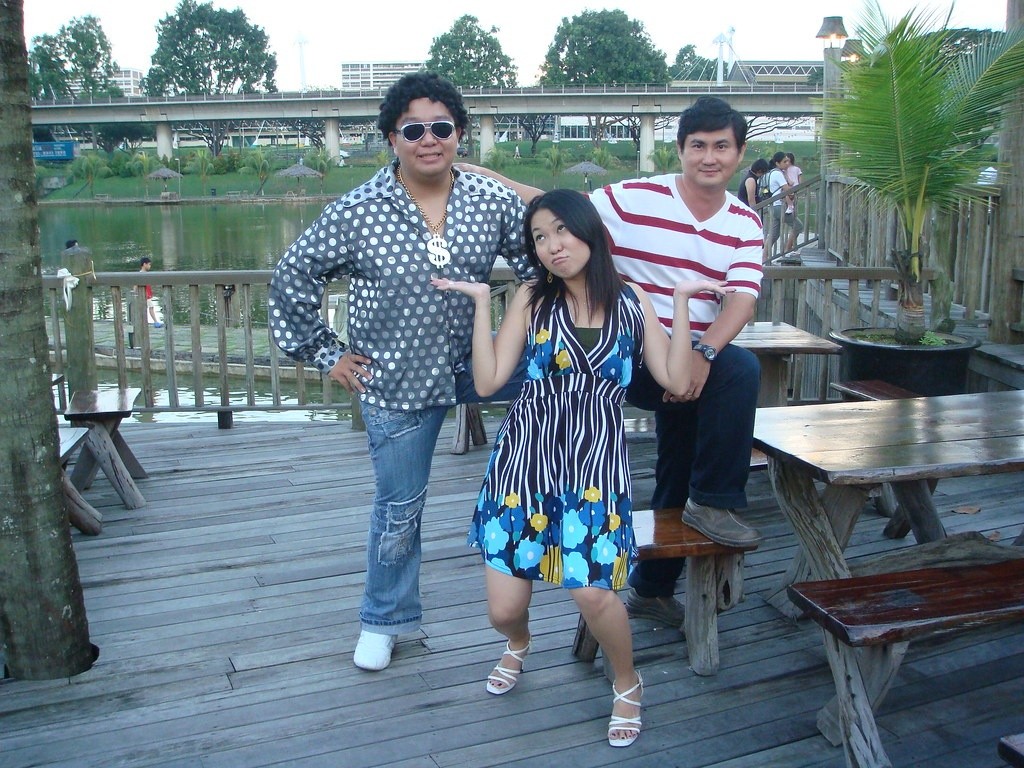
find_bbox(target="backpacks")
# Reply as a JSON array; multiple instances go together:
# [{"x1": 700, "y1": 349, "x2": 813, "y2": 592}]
[{"x1": 759, "y1": 169, "x2": 782, "y2": 201}]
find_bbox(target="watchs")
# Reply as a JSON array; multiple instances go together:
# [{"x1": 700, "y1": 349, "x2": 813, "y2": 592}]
[{"x1": 693, "y1": 343, "x2": 718, "y2": 362}]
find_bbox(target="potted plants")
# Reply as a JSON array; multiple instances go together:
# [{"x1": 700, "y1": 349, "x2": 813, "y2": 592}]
[{"x1": 806, "y1": 1, "x2": 1024, "y2": 403}]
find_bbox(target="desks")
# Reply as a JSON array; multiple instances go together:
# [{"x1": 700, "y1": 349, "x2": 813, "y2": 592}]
[
  {"x1": 729, "y1": 319, "x2": 844, "y2": 408},
  {"x1": 752, "y1": 389, "x2": 1024, "y2": 622}
]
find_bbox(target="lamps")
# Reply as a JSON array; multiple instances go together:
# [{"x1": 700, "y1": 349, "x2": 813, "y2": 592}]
[
  {"x1": 842, "y1": 39, "x2": 868, "y2": 62},
  {"x1": 816, "y1": 16, "x2": 848, "y2": 48}
]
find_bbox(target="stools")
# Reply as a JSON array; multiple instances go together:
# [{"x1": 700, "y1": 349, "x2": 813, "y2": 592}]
[
  {"x1": 58, "y1": 427, "x2": 104, "y2": 536},
  {"x1": 64, "y1": 388, "x2": 149, "y2": 509},
  {"x1": 568, "y1": 506, "x2": 758, "y2": 677}
]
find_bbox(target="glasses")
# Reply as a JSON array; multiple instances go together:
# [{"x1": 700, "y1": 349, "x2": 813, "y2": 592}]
[{"x1": 394, "y1": 121, "x2": 455, "y2": 143}]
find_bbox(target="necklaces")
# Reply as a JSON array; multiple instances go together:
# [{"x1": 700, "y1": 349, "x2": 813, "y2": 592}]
[{"x1": 396, "y1": 162, "x2": 454, "y2": 268}]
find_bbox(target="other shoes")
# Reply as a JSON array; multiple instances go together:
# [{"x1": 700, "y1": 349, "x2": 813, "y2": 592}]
[
  {"x1": 785, "y1": 205, "x2": 794, "y2": 214},
  {"x1": 784, "y1": 250, "x2": 800, "y2": 257}
]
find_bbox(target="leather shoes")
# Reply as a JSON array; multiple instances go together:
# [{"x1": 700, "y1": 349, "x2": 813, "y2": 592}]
[
  {"x1": 681, "y1": 497, "x2": 765, "y2": 547},
  {"x1": 625, "y1": 588, "x2": 686, "y2": 627}
]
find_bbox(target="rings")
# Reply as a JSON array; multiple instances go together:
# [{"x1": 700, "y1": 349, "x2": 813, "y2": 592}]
[{"x1": 687, "y1": 393, "x2": 693, "y2": 396}]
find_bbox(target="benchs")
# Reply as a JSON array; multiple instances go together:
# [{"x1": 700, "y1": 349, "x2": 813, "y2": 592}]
[
  {"x1": 624, "y1": 417, "x2": 768, "y2": 471},
  {"x1": 828, "y1": 379, "x2": 927, "y2": 517},
  {"x1": 788, "y1": 558, "x2": 1024, "y2": 768}
]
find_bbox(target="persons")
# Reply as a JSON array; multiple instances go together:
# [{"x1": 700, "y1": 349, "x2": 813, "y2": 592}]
[
  {"x1": 977, "y1": 158, "x2": 997, "y2": 213},
  {"x1": 513, "y1": 144, "x2": 520, "y2": 158},
  {"x1": 133, "y1": 257, "x2": 164, "y2": 328},
  {"x1": 449, "y1": 94, "x2": 764, "y2": 632},
  {"x1": 783, "y1": 152, "x2": 803, "y2": 215},
  {"x1": 738, "y1": 159, "x2": 768, "y2": 250},
  {"x1": 462, "y1": 147, "x2": 468, "y2": 158},
  {"x1": 762, "y1": 152, "x2": 804, "y2": 264},
  {"x1": 65, "y1": 240, "x2": 79, "y2": 248},
  {"x1": 430, "y1": 188, "x2": 739, "y2": 750},
  {"x1": 269, "y1": 69, "x2": 539, "y2": 671}
]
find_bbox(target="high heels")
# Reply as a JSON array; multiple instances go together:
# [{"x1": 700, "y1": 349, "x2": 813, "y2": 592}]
[
  {"x1": 487, "y1": 631, "x2": 531, "y2": 695},
  {"x1": 607, "y1": 670, "x2": 643, "y2": 748}
]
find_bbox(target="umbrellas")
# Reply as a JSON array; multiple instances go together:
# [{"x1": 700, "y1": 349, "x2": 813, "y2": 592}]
[
  {"x1": 563, "y1": 161, "x2": 608, "y2": 190},
  {"x1": 275, "y1": 163, "x2": 323, "y2": 192},
  {"x1": 147, "y1": 167, "x2": 182, "y2": 190}
]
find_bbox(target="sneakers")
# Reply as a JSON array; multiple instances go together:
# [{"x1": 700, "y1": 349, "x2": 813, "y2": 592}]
[{"x1": 354, "y1": 630, "x2": 397, "y2": 671}]
